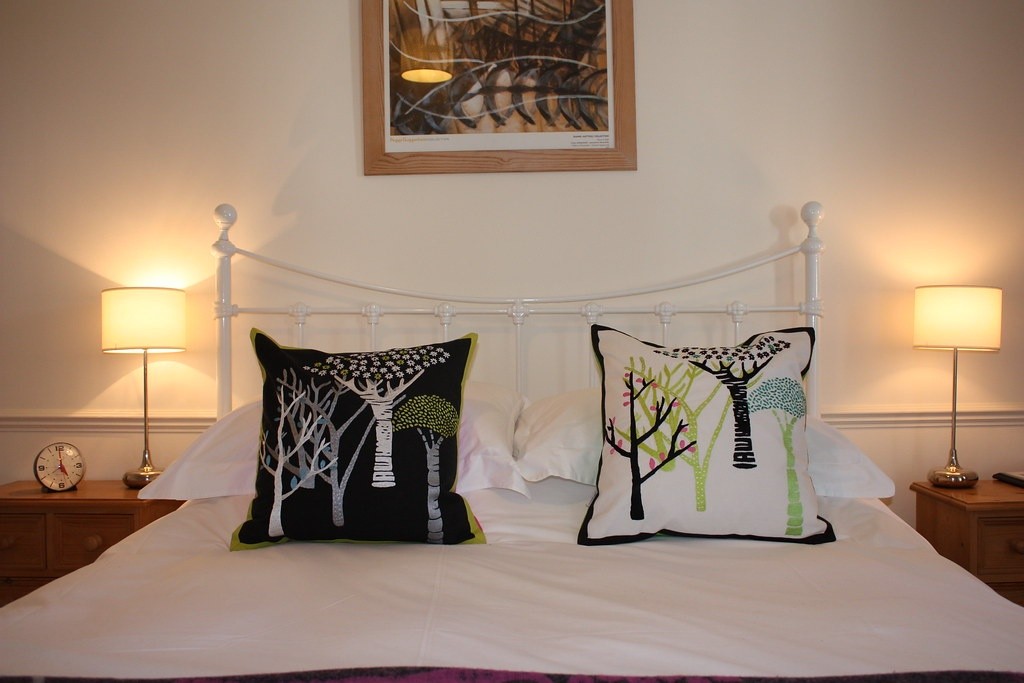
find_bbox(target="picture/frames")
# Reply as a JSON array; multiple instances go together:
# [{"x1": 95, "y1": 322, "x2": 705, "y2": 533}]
[{"x1": 361, "y1": 0, "x2": 638, "y2": 177}]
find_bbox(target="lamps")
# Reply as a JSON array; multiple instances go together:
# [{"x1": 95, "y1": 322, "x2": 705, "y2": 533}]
[
  {"x1": 101, "y1": 286, "x2": 187, "y2": 488},
  {"x1": 911, "y1": 286, "x2": 1002, "y2": 487},
  {"x1": 399, "y1": 26, "x2": 453, "y2": 84}
]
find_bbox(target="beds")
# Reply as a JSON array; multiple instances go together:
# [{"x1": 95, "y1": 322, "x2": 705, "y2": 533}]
[{"x1": 0, "y1": 200, "x2": 1024, "y2": 683}]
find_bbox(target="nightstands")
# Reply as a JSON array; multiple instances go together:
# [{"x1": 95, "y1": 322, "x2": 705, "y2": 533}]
[
  {"x1": 909, "y1": 480, "x2": 1024, "y2": 607},
  {"x1": 0, "y1": 479, "x2": 186, "y2": 607}
]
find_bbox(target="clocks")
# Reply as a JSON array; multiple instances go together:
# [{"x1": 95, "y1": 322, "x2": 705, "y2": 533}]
[{"x1": 33, "y1": 442, "x2": 86, "y2": 493}]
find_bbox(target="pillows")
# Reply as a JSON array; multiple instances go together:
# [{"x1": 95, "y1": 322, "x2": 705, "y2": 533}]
[
  {"x1": 228, "y1": 328, "x2": 487, "y2": 546},
  {"x1": 137, "y1": 381, "x2": 530, "y2": 500},
  {"x1": 514, "y1": 384, "x2": 897, "y2": 498},
  {"x1": 577, "y1": 325, "x2": 836, "y2": 546}
]
